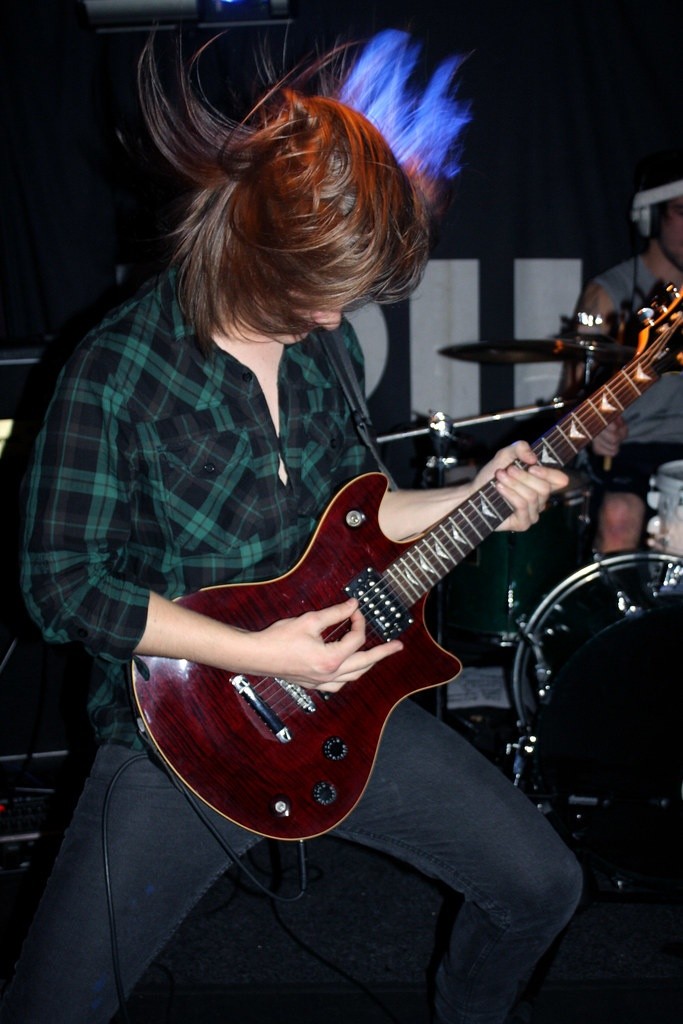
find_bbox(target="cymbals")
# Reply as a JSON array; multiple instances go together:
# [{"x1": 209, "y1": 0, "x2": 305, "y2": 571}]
[{"x1": 437, "y1": 330, "x2": 681, "y2": 367}]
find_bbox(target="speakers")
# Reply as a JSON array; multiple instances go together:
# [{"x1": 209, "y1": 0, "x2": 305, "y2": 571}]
[{"x1": 0, "y1": 344, "x2": 100, "y2": 872}]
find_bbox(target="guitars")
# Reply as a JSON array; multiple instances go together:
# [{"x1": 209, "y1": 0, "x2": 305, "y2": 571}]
[{"x1": 126, "y1": 282, "x2": 683, "y2": 842}]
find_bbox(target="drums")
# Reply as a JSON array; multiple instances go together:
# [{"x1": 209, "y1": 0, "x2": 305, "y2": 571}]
[
  {"x1": 645, "y1": 460, "x2": 683, "y2": 557},
  {"x1": 511, "y1": 547, "x2": 683, "y2": 739}
]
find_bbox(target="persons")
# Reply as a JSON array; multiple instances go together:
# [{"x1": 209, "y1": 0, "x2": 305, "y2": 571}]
[
  {"x1": 570, "y1": 146, "x2": 683, "y2": 565},
  {"x1": 0, "y1": 17, "x2": 586, "y2": 1024}
]
